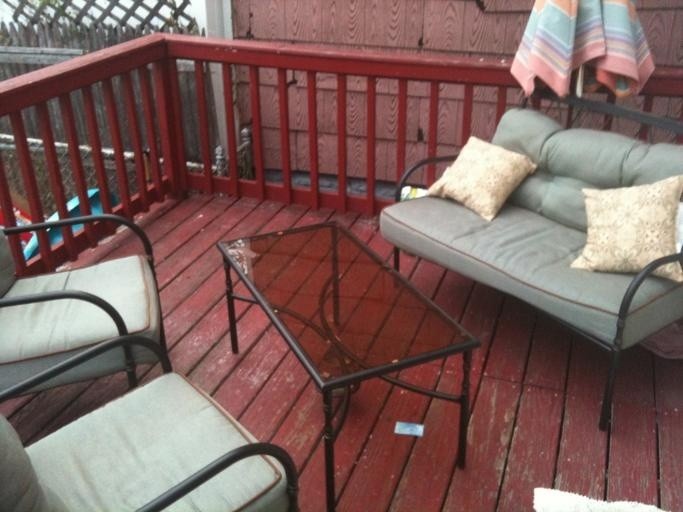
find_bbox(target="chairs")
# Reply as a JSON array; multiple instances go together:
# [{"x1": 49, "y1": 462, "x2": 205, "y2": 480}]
[
  {"x1": 0, "y1": 335, "x2": 298, "y2": 511},
  {"x1": 0, "y1": 214, "x2": 172, "y2": 388}
]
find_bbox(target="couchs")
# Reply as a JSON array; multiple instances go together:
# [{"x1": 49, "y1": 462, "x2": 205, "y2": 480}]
[{"x1": 379, "y1": 88, "x2": 683, "y2": 432}]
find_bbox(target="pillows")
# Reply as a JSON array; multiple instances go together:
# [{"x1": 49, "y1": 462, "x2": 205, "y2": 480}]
[
  {"x1": 570, "y1": 175, "x2": 683, "y2": 282},
  {"x1": 425, "y1": 136, "x2": 538, "y2": 222}
]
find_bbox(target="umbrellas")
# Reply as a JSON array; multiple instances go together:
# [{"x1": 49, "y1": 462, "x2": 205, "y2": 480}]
[{"x1": 509, "y1": 0, "x2": 654, "y2": 104}]
